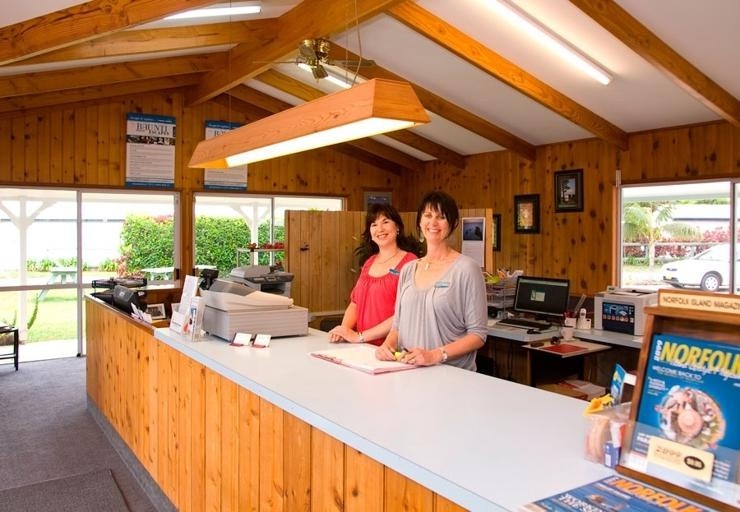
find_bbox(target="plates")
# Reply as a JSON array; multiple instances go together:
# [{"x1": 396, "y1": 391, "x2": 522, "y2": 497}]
[{"x1": 559, "y1": 339, "x2": 577, "y2": 342}]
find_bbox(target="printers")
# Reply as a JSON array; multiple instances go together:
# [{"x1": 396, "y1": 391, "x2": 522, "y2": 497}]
[{"x1": 228, "y1": 264, "x2": 294, "y2": 298}]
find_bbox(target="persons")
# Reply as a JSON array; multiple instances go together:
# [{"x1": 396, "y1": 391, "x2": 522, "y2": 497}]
[
  {"x1": 327, "y1": 200, "x2": 417, "y2": 346},
  {"x1": 375, "y1": 189, "x2": 488, "y2": 369}
]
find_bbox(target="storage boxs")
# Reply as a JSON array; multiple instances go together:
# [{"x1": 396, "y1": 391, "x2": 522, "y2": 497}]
[{"x1": 594, "y1": 292, "x2": 658, "y2": 336}]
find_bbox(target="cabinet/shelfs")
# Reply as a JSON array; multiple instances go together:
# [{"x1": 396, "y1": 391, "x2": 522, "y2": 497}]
[{"x1": 85, "y1": 294, "x2": 740, "y2": 512}]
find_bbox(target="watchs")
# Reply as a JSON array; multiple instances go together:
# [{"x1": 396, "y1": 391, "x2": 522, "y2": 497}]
[
  {"x1": 357, "y1": 332, "x2": 362, "y2": 342},
  {"x1": 440, "y1": 349, "x2": 448, "y2": 365}
]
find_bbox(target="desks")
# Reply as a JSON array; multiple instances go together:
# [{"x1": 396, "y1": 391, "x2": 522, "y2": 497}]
[{"x1": 236, "y1": 247, "x2": 304, "y2": 267}]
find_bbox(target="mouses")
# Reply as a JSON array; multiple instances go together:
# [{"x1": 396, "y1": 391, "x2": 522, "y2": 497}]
[{"x1": 526, "y1": 329, "x2": 541, "y2": 335}]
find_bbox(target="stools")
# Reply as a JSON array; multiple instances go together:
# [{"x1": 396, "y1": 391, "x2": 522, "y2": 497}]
[{"x1": 0, "y1": 327, "x2": 20, "y2": 371}]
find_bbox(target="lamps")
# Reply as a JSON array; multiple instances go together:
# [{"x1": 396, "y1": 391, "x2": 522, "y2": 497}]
[
  {"x1": 485, "y1": 0, "x2": 615, "y2": 89},
  {"x1": 164, "y1": 5, "x2": 262, "y2": 21},
  {"x1": 188, "y1": 78, "x2": 429, "y2": 168},
  {"x1": 298, "y1": 38, "x2": 331, "y2": 80}
]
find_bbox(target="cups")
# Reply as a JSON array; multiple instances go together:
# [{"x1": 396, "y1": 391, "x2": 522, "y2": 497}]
[
  {"x1": 565, "y1": 317, "x2": 576, "y2": 329},
  {"x1": 560, "y1": 325, "x2": 574, "y2": 339}
]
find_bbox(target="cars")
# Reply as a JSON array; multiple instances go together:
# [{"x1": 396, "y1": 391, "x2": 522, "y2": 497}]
[{"x1": 661, "y1": 243, "x2": 740, "y2": 292}]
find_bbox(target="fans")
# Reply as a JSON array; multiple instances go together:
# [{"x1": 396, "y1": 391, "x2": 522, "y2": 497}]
[{"x1": 253, "y1": 38, "x2": 377, "y2": 70}]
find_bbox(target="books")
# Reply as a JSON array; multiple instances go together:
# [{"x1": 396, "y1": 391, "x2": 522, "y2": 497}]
[
  {"x1": 311, "y1": 341, "x2": 415, "y2": 375},
  {"x1": 629, "y1": 331, "x2": 739, "y2": 501}
]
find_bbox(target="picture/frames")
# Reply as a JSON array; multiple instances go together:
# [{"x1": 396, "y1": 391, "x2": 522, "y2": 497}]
[
  {"x1": 554, "y1": 168, "x2": 584, "y2": 212},
  {"x1": 513, "y1": 194, "x2": 540, "y2": 235}
]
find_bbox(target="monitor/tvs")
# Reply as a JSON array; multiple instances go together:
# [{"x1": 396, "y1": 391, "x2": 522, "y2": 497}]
[
  {"x1": 513, "y1": 275, "x2": 570, "y2": 320},
  {"x1": 111, "y1": 285, "x2": 145, "y2": 316}
]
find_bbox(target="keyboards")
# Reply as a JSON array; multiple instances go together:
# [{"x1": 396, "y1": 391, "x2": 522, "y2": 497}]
[{"x1": 497, "y1": 318, "x2": 552, "y2": 331}]
